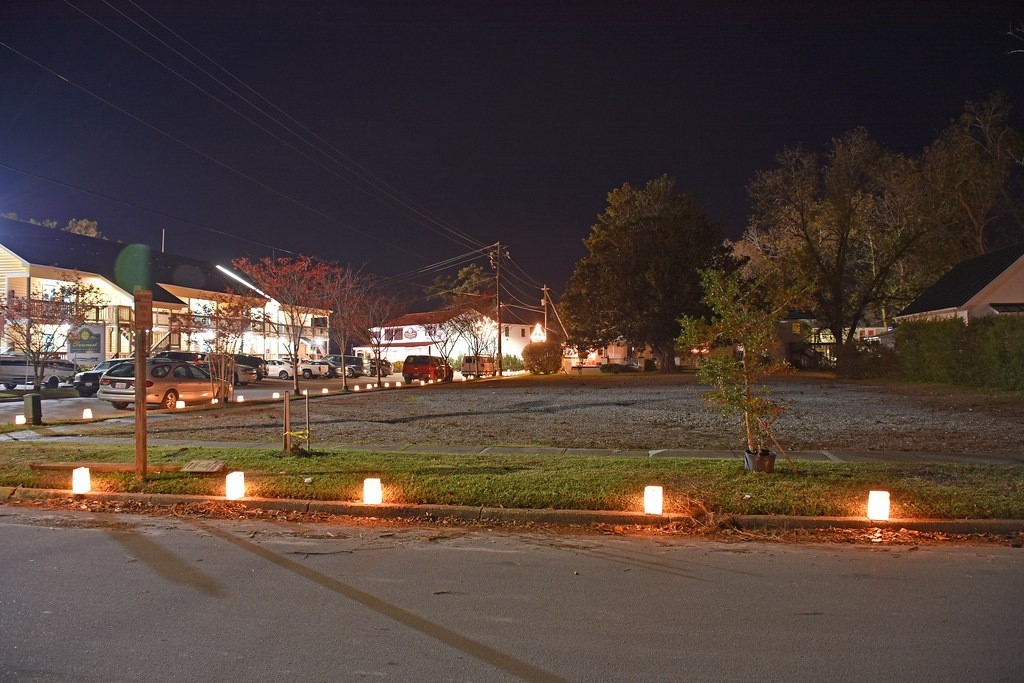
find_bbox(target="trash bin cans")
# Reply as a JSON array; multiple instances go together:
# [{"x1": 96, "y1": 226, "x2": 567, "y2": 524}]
[{"x1": 23, "y1": 393, "x2": 43, "y2": 424}]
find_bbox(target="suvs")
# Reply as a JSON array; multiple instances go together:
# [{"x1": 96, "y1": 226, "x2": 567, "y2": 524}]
[
  {"x1": 209, "y1": 354, "x2": 257, "y2": 385},
  {"x1": 74, "y1": 358, "x2": 171, "y2": 397},
  {"x1": 369, "y1": 359, "x2": 394, "y2": 377},
  {"x1": 362, "y1": 359, "x2": 372, "y2": 377},
  {"x1": 229, "y1": 355, "x2": 268, "y2": 382}
]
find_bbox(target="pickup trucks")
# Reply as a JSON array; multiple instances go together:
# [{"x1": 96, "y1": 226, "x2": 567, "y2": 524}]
[
  {"x1": 402, "y1": 355, "x2": 453, "y2": 385},
  {"x1": 283, "y1": 357, "x2": 329, "y2": 380},
  {"x1": 151, "y1": 351, "x2": 210, "y2": 375}
]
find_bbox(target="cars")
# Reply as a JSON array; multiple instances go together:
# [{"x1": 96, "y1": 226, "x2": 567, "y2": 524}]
[
  {"x1": 314, "y1": 360, "x2": 343, "y2": 378},
  {"x1": 97, "y1": 361, "x2": 233, "y2": 409},
  {"x1": 0, "y1": 347, "x2": 80, "y2": 388},
  {"x1": 266, "y1": 360, "x2": 295, "y2": 379}
]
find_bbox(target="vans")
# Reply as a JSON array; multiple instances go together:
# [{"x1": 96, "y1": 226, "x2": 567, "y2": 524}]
[
  {"x1": 321, "y1": 356, "x2": 364, "y2": 378},
  {"x1": 461, "y1": 354, "x2": 497, "y2": 378}
]
